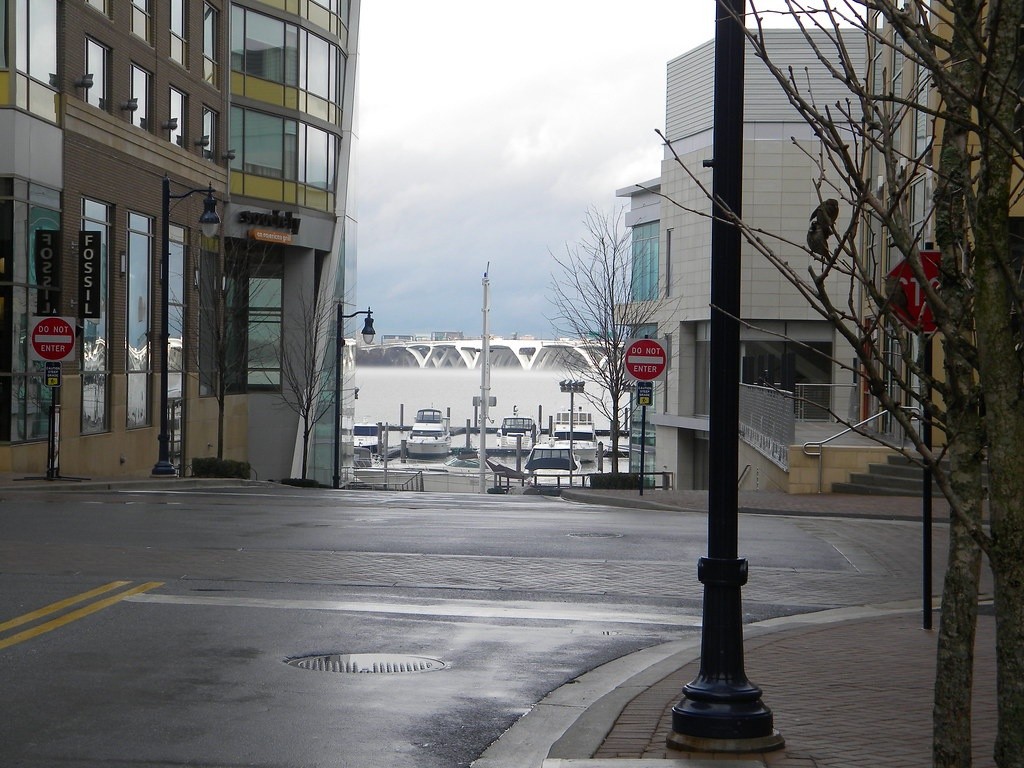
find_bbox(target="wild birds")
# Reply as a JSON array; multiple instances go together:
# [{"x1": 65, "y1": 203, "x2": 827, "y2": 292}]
[{"x1": 807, "y1": 198, "x2": 839, "y2": 266}]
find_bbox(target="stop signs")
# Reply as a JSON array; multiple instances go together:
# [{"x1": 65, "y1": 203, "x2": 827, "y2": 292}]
[
  {"x1": 624, "y1": 338, "x2": 667, "y2": 382},
  {"x1": 885, "y1": 248, "x2": 949, "y2": 336},
  {"x1": 27, "y1": 316, "x2": 75, "y2": 364}
]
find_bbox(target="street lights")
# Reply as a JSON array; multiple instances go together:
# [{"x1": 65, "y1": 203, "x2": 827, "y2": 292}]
[
  {"x1": 150, "y1": 170, "x2": 222, "y2": 478},
  {"x1": 332, "y1": 303, "x2": 375, "y2": 490},
  {"x1": 559, "y1": 379, "x2": 586, "y2": 489}
]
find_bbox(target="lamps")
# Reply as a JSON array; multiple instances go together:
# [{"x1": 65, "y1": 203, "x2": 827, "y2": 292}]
[
  {"x1": 222, "y1": 148, "x2": 236, "y2": 160},
  {"x1": 163, "y1": 117, "x2": 178, "y2": 131},
  {"x1": 75, "y1": 74, "x2": 94, "y2": 89},
  {"x1": 120, "y1": 97, "x2": 139, "y2": 111},
  {"x1": 195, "y1": 135, "x2": 210, "y2": 146}
]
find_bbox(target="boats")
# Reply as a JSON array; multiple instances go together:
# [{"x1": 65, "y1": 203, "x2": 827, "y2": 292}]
[
  {"x1": 352, "y1": 424, "x2": 380, "y2": 453},
  {"x1": 551, "y1": 407, "x2": 597, "y2": 464},
  {"x1": 496, "y1": 404, "x2": 539, "y2": 450},
  {"x1": 407, "y1": 409, "x2": 452, "y2": 460},
  {"x1": 522, "y1": 437, "x2": 582, "y2": 486}
]
